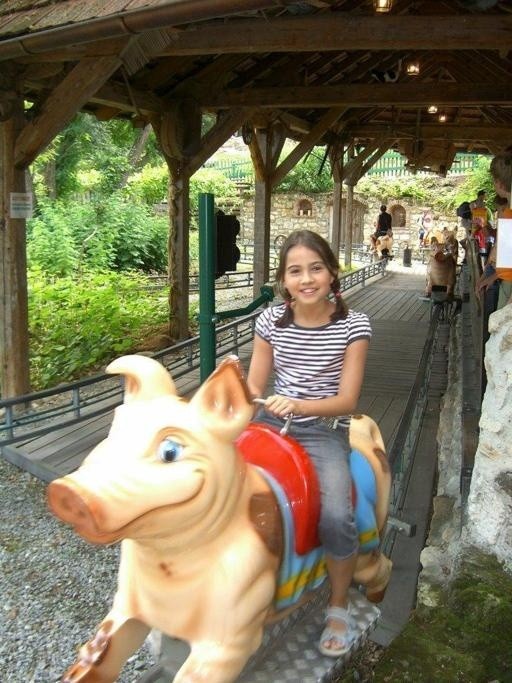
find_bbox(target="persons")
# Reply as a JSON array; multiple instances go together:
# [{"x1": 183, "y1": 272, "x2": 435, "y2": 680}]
[
  {"x1": 456, "y1": 145, "x2": 512, "y2": 311},
  {"x1": 246, "y1": 229, "x2": 372, "y2": 657},
  {"x1": 370, "y1": 205, "x2": 392, "y2": 250}
]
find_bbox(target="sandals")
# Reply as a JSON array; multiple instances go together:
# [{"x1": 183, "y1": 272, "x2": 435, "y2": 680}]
[{"x1": 317, "y1": 600, "x2": 357, "y2": 659}]
